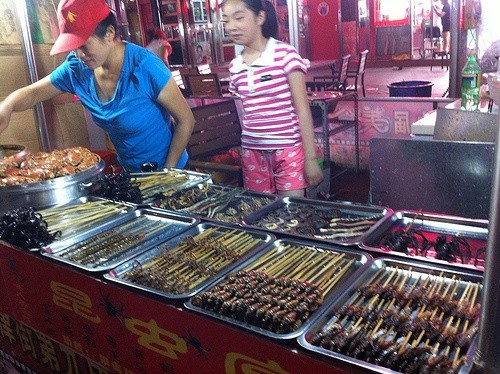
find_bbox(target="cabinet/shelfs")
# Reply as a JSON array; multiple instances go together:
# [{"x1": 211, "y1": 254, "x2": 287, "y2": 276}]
[{"x1": 310, "y1": 91, "x2": 360, "y2": 173}]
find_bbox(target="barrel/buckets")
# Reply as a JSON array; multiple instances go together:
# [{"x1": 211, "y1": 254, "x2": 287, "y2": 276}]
[{"x1": 387, "y1": 80, "x2": 434, "y2": 97}]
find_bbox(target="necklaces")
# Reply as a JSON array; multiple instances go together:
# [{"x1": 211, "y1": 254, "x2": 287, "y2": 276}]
[{"x1": 91, "y1": 76, "x2": 112, "y2": 99}]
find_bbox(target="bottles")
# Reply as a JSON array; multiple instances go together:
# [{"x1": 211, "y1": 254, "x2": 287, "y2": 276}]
[{"x1": 460, "y1": 54, "x2": 481, "y2": 112}]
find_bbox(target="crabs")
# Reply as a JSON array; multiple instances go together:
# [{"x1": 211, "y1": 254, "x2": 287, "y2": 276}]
[{"x1": 0, "y1": 146, "x2": 103, "y2": 186}]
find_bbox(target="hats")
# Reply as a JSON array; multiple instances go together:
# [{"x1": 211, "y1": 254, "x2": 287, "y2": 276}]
[
  {"x1": 154, "y1": 29, "x2": 166, "y2": 38},
  {"x1": 49, "y1": 0, "x2": 110, "y2": 57}
]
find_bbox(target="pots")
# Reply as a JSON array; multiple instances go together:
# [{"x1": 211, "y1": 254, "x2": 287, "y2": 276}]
[{"x1": 0, "y1": 158, "x2": 107, "y2": 213}]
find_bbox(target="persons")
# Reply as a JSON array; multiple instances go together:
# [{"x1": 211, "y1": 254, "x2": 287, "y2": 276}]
[
  {"x1": 434, "y1": 0, "x2": 451, "y2": 56},
  {"x1": 0, "y1": 0, "x2": 198, "y2": 173},
  {"x1": 216, "y1": 0, "x2": 326, "y2": 198},
  {"x1": 196, "y1": 45, "x2": 207, "y2": 64}
]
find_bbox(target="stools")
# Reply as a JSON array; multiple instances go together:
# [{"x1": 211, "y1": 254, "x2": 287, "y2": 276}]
[{"x1": 431, "y1": 50, "x2": 449, "y2": 71}]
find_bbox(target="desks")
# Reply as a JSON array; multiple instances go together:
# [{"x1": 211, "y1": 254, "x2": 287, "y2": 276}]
[
  {"x1": 213, "y1": 60, "x2": 336, "y2": 81},
  {"x1": 412, "y1": 99, "x2": 500, "y2": 135}
]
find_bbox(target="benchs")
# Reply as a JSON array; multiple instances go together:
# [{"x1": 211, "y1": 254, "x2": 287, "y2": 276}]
[{"x1": 188, "y1": 100, "x2": 243, "y2": 188}]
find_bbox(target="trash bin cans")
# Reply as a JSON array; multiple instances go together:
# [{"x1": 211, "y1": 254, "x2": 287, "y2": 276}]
[{"x1": 387, "y1": 81, "x2": 434, "y2": 97}]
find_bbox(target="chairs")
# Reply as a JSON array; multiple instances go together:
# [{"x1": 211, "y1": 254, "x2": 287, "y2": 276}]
[
  {"x1": 313, "y1": 49, "x2": 369, "y2": 97},
  {"x1": 172, "y1": 63, "x2": 222, "y2": 96}
]
